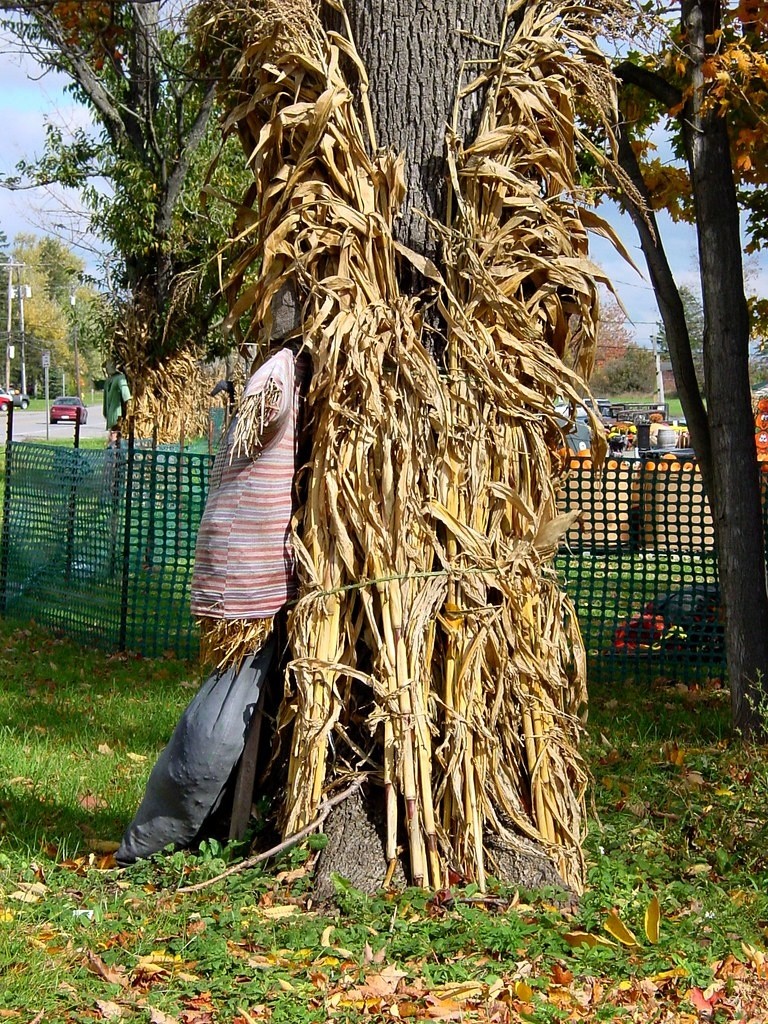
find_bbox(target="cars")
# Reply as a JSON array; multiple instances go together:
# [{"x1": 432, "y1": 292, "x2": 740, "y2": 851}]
[
  {"x1": 50, "y1": 397, "x2": 88, "y2": 425},
  {"x1": 0, "y1": 383, "x2": 33, "y2": 411}
]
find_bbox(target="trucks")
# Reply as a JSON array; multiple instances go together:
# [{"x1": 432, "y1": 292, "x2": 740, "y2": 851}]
[{"x1": 555, "y1": 399, "x2": 698, "y2": 460}]
